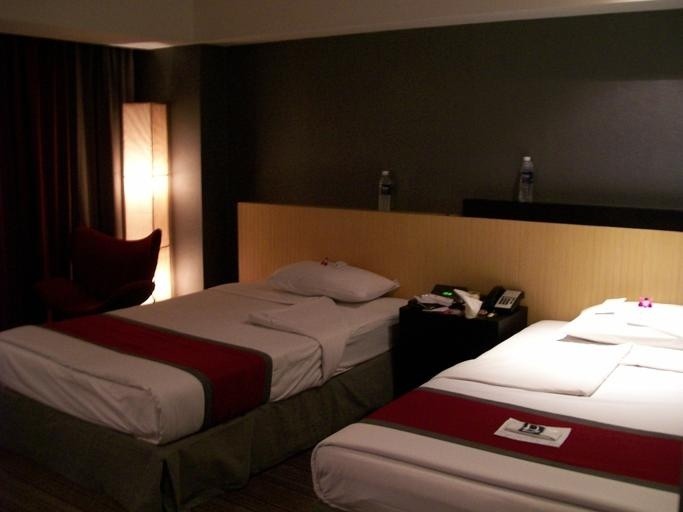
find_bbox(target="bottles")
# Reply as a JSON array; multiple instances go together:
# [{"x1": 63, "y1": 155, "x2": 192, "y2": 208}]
[
  {"x1": 517, "y1": 155, "x2": 535, "y2": 203},
  {"x1": 377, "y1": 170, "x2": 392, "y2": 213}
]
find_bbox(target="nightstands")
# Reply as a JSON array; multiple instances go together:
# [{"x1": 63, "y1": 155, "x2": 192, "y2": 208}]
[{"x1": 399, "y1": 303, "x2": 528, "y2": 394}]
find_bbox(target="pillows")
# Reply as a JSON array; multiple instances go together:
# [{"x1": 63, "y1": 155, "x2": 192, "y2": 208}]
[
  {"x1": 556, "y1": 303, "x2": 683, "y2": 351},
  {"x1": 266, "y1": 260, "x2": 399, "y2": 302}
]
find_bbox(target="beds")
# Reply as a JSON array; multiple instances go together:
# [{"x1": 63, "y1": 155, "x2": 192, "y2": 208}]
[
  {"x1": 0, "y1": 281, "x2": 407, "y2": 512},
  {"x1": 309, "y1": 320, "x2": 683, "y2": 512}
]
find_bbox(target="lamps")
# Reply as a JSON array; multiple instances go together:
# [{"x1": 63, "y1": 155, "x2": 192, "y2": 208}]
[{"x1": 121, "y1": 103, "x2": 171, "y2": 302}]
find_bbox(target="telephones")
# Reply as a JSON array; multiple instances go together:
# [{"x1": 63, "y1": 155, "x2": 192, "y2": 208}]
[{"x1": 484, "y1": 286, "x2": 524, "y2": 314}]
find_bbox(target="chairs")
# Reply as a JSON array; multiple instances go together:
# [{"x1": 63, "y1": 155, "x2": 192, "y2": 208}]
[{"x1": 37, "y1": 222, "x2": 161, "y2": 320}]
